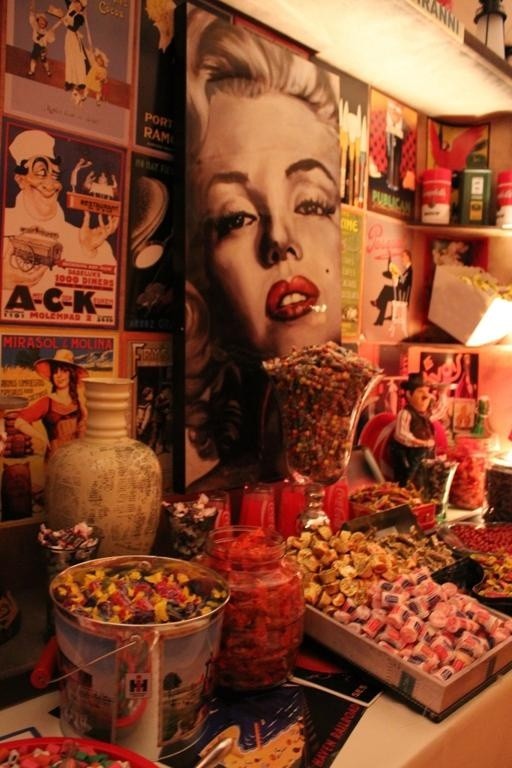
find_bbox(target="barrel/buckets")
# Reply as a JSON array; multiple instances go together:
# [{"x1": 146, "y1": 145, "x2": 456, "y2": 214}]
[{"x1": 45, "y1": 553, "x2": 234, "y2": 760}]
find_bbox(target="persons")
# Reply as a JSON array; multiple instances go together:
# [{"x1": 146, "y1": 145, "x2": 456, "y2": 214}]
[
  {"x1": 388, "y1": 255, "x2": 401, "y2": 300},
  {"x1": 13, "y1": 349, "x2": 90, "y2": 463},
  {"x1": 369, "y1": 251, "x2": 411, "y2": 326},
  {"x1": 392, "y1": 374, "x2": 447, "y2": 502},
  {"x1": 184, "y1": 11, "x2": 342, "y2": 495}
]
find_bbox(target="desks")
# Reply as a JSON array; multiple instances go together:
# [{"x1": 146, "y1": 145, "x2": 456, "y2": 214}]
[{"x1": 0, "y1": 618, "x2": 510, "y2": 768}]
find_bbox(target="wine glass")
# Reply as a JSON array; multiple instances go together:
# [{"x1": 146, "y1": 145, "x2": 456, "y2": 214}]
[{"x1": 263, "y1": 358, "x2": 385, "y2": 538}]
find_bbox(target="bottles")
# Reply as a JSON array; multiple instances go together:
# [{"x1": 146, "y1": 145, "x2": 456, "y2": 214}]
[
  {"x1": 191, "y1": 526, "x2": 304, "y2": 689},
  {"x1": 495, "y1": 170, "x2": 512, "y2": 228},
  {"x1": 422, "y1": 165, "x2": 452, "y2": 224},
  {"x1": 471, "y1": 398, "x2": 493, "y2": 437}
]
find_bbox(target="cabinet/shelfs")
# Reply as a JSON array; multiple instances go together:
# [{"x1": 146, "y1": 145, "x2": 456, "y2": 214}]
[{"x1": 220, "y1": 0, "x2": 512, "y2": 354}]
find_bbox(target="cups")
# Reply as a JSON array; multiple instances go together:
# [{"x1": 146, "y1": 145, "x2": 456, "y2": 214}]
[
  {"x1": 418, "y1": 457, "x2": 462, "y2": 522},
  {"x1": 198, "y1": 471, "x2": 353, "y2": 541},
  {"x1": 43, "y1": 526, "x2": 100, "y2": 639}
]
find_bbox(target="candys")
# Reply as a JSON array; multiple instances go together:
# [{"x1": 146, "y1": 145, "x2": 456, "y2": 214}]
[
  {"x1": 38, "y1": 520, "x2": 99, "y2": 553},
  {"x1": 1, "y1": 739, "x2": 130, "y2": 767},
  {"x1": 449, "y1": 266, "x2": 512, "y2": 307},
  {"x1": 163, "y1": 493, "x2": 218, "y2": 555},
  {"x1": 191, "y1": 528, "x2": 306, "y2": 688},
  {"x1": 279, "y1": 522, "x2": 512, "y2": 686},
  {"x1": 457, "y1": 452, "x2": 488, "y2": 511},
  {"x1": 348, "y1": 479, "x2": 423, "y2": 515},
  {"x1": 422, "y1": 457, "x2": 460, "y2": 507},
  {"x1": 263, "y1": 339, "x2": 385, "y2": 486},
  {"x1": 54, "y1": 558, "x2": 226, "y2": 625}
]
